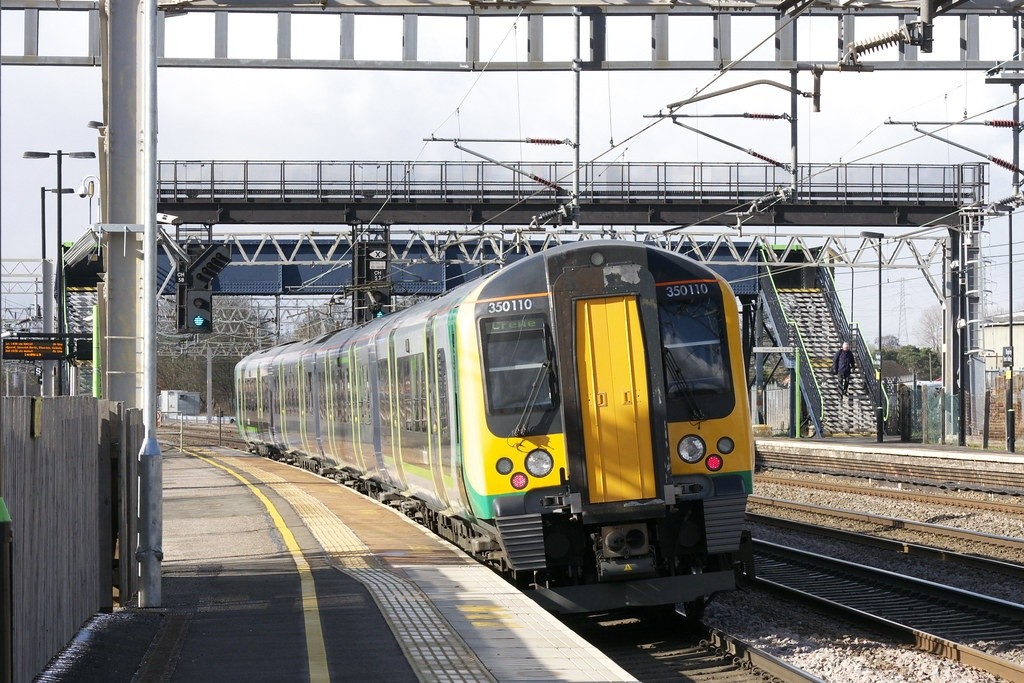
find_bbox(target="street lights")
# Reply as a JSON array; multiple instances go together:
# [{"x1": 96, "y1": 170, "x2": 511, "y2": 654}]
[{"x1": 21, "y1": 149, "x2": 97, "y2": 395}]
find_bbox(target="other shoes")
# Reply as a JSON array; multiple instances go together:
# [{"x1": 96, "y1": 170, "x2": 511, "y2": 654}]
[
  {"x1": 838, "y1": 395, "x2": 843, "y2": 400},
  {"x1": 845, "y1": 392, "x2": 849, "y2": 397}
]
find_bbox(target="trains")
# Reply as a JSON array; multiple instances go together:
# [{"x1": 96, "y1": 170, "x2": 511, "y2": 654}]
[{"x1": 235, "y1": 238, "x2": 755, "y2": 626}]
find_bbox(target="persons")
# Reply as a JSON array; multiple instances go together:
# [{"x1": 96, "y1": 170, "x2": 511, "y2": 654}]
[{"x1": 833, "y1": 342, "x2": 855, "y2": 402}]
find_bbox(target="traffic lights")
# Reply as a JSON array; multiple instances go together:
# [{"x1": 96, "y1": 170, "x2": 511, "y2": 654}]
[{"x1": 186, "y1": 288, "x2": 212, "y2": 334}]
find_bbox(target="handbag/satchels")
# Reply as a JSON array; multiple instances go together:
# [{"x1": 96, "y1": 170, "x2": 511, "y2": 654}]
[{"x1": 829, "y1": 365, "x2": 838, "y2": 375}]
